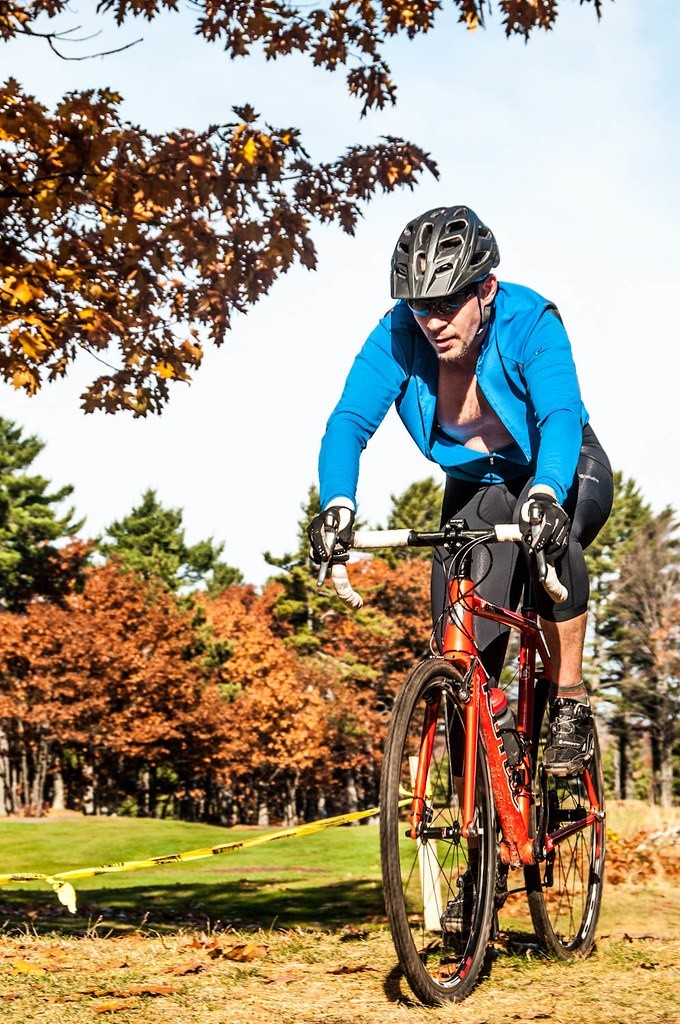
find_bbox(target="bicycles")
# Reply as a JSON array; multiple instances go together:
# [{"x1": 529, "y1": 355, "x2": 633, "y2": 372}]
[{"x1": 312, "y1": 498, "x2": 608, "y2": 1008}]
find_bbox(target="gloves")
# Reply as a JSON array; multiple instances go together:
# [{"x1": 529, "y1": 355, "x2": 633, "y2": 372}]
[
  {"x1": 306, "y1": 505, "x2": 355, "y2": 568},
  {"x1": 519, "y1": 493, "x2": 570, "y2": 567}
]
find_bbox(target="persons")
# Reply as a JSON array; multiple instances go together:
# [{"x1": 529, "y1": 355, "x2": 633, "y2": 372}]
[{"x1": 305, "y1": 206, "x2": 613, "y2": 934}]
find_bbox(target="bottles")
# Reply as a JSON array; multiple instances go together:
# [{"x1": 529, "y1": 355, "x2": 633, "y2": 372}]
[{"x1": 487, "y1": 688, "x2": 522, "y2": 765}]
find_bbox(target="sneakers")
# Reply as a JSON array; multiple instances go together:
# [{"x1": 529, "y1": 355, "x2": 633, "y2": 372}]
[
  {"x1": 541, "y1": 695, "x2": 595, "y2": 778},
  {"x1": 440, "y1": 861, "x2": 508, "y2": 933}
]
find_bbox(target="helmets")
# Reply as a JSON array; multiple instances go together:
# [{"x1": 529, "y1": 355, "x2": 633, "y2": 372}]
[{"x1": 390, "y1": 205, "x2": 500, "y2": 300}]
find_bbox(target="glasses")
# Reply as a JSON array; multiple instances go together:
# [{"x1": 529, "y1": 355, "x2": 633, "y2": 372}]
[{"x1": 405, "y1": 283, "x2": 480, "y2": 318}]
